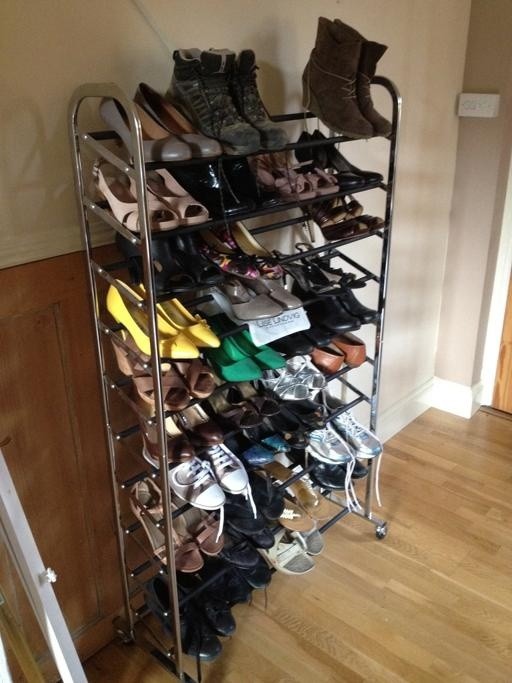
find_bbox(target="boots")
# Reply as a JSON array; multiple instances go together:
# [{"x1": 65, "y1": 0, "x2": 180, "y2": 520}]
[
  {"x1": 357, "y1": 41, "x2": 393, "y2": 137},
  {"x1": 208, "y1": 47, "x2": 288, "y2": 151},
  {"x1": 302, "y1": 16, "x2": 375, "y2": 139},
  {"x1": 164, "y1": 47, "x2": 261, "y2": 154}
]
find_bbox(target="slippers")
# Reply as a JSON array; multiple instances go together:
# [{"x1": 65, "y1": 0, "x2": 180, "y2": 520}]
[
  {"x1": 278, "y1": 496, "x2": 313, "y2": 532},
  {"x1": 218, "y1": 494, "x2": 266, "y2": 535},
  {"x1": 249, "y1": 469, "x2": 285, "y2": 520},
  {"x1": 288, "y1": 522, "x2": 326, "y2": 555},
  {"x1": 265, "y1": 461, "x2": 330, "y2": 521},
  {"x1": 258, "y1": 529, "x2": 316, "y2": 575}
]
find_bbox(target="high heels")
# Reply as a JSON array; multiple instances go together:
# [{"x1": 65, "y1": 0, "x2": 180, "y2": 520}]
[
  {"x1": 295, "y1": 242, "x2": 366, "y2": 288},
  {"x1": 271, "y1": 249, "x2": 347, "y2": 296},
  {"x1": 175, "y1": 358, "x2": 216, "y2": 399},
  {"x1": 300, "y1": 192, "x2": 385, "y2": 244},
  {"x1": 111, "y1": 328, "x2": 191, "y2": 411}
]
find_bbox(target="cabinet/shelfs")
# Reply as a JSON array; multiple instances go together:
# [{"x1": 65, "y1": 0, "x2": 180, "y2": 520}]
[{"x1": 66, "y1": 70, "x2": 403, "y2": 682}]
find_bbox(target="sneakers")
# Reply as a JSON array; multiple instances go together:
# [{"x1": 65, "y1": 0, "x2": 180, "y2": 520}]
[
  {"x1": 195, "y1": 437, "x2": 249, "y2": 493},
  {"x1": 142, "y1": 449, "x2": 226, "y2": 509},
  {"x1": 315, "y1": 389, "x2": 382, "y2": 459},
  {"x1": 304, "y1": 421, "x2": 355, "y2": 465}
]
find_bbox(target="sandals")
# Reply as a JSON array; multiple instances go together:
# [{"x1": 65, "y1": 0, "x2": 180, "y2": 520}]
[
  {"x1": 233, "y1": 381, "x2": 281, "y2": 417},
  {"x1": 143, "y1": 168, "x2": 209, "y2": 225},
  {"x1": 208, "y1": 384, "x2": 263, "y2": 429},
  {"x1": 252, "y1": 366, "x2": 310, "y2": 400},
  {"x1": 170, "y1": 489, "x2": 225, "y2": 555},
  {"x1": 127, "y1": 477, "x2": 204, "y2": 573},
  {"x1": 285, "y1": 354, "x2": 325, "y2": 389},
  {"x1": 92, "y1": 156, "x2": 180, "y2": 233},
  {"x1": 254, "y1": 149, "x2": 340, "y2": 201}
]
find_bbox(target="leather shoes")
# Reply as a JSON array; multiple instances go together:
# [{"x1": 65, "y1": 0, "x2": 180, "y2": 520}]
[
  {"x1": 272, "y1": 332, "x2": 312, "y2": 354},
  {"x1": 244, "y1": 424, "x2": 291, "y2": 453},
  {"x1": 144, "y1": 576, "x2": 222, "y2": 659},
  {"x1": 305, "y1": 293, "x2": 361, "y2": 331},
  {"x1": 312, "y1": 130, "x2": 383, "y2": 188},
  {"x1": 219, "y1": 526, "x2": 260, "y2": 569},
  {"x1": 140, "y1": 416, "x2": 195, "y2": 461},
  {"x1": 295, "y1": 131, "x2": 366, "y2": 190},
  {"x1": 172, "y1": 162, "x2": 256, "y2": 217},
  {"x1": 253, "y1": 275, "x2": 303, "y2": 310},
  {"x1": 137, "y1": 282, "x2": 221, "y2": 349},
  {"x1": 335, "y1": 286, "x2": 379, "y2": 323},
  {"x1": 220, "y1": 159, "x2": 281, "y2": 208},
  {"x1": 174, "y1": 403, "x2": 224, "y2": 445},
  {"x1": 222, "y1": 220, "x2": 283, "y2": 279},
  {"x1": 206, "y1": 336, "x2": 264, "y2": 380},
  {"x1": 237, "y1": 558, "x2": 272, "y2": 589},
  {"x1": 344, "y1": 460, "x2": 369, "y2": 478},
  {"x1": 235, "y1": 329, "x2": 287, "y2": 369},
  {"x1": 306, "y1": 324, "x2": 331, "y2": 347},
  {"x1": 106, "y1": 278, "x2": 200, "y2": 361},
  {"x1": 308, "y1": 343, "x2": 345, "y2": 375},
  {"x1": 162, "y1": 563, "x2": 237, "y2": 637},
  {"x1": 227, "y1": 517, "x2": 275, "y2": 547},
  {"x1": 115, "y1": 235, "x2": 197, "y2": 293},
  {"x1": 332, "y1": 333, "x2": 366, "y2": 367},
  {"x1": 269, "y1": 416, "x2": 310, "y2": 449},
  {"x1": 195, "y1": 228, "x2": 261, "y2": 280},
  {"x1": 99, "y1": 96, "x2": 192, "y2": 162},
  {"x1": 197, "y1": 558, "x2": 252, "y2": 603},
  {"x1": 134, "y1": 82, "x2": 222, "y2": 157},
  {"x1": 228, "y1": 434, "x2": 275, "y2": 465},
  {"x1": 286, "y1": 451, "x2": 355, "y2": 490},
  {"x1": 287, "y1": 403, "x2": 326, "y2": 429},
  {"x1": 171, "y1": 234, "x2": 225, "y2": 284},
  {"x1": 220, "y1": 279, "x2": 284, "y2": 319}
]
